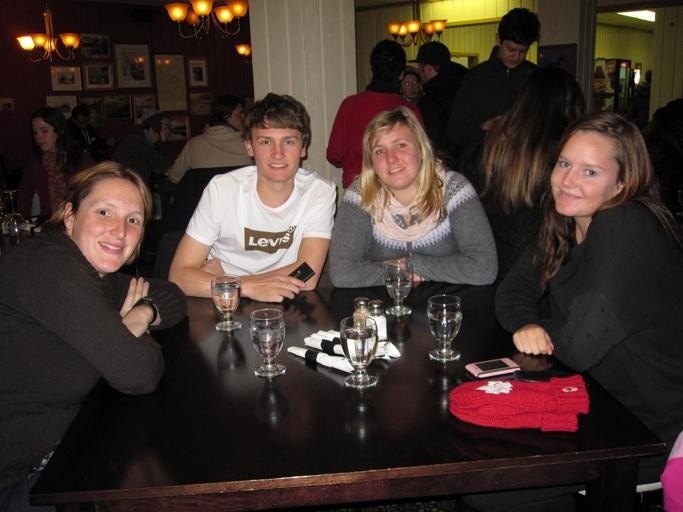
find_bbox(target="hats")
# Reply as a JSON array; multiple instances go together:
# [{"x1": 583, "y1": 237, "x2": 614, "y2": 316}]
[
  {"x1": 408, "y1": 41, "x2": 450, "y2": 66},
  {"x1": 141, "y1": 111, "x2": 168, "y2": 132}
]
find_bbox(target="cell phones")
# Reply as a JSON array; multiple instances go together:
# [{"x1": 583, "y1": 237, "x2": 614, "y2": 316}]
[
  {"x1": 465, "y1": 357, "x2": 521, "y2": 379},
  {"x1": 287, "y1": 262, "x2": 315, "y2": 283}
]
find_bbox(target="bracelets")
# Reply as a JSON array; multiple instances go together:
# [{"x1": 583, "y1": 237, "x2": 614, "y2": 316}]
[{"x1": 132, "y1": 297, "x2": 157, "y2": 322}]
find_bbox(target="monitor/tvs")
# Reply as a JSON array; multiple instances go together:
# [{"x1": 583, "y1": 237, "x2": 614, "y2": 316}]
[{"x1": 536, "y1": 42, "x2": 577, "y2": 81}]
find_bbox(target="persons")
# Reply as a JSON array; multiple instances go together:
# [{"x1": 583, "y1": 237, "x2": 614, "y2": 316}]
[
  {"x1": 447, "y1": 7, "x2": 541, "y2": 200},
  {"x1": 481, "y1": 65, "x2": 585, "y2": 274},
  {"x1": 17, "y1": 93, "x2": 256, "y2": 225},
  {"x1": 625, "y1": 69, "x2": 652, "y2": 132},
  {"x1": 461, "y1": 112, "x2": 683, "y2": 511},
  {"x1": 165, "y1": 93, "x2": 336, "y2": 304},
  {"x1": 399, "y1": 40, "x2": 469, "y2": 157},
  {"x1": 643, "y1": 97, "x2": 680, "y2": 229},
  {"x1": 0, "y1": 162, "x2": 189, "y2": 511},
  {"x1": 326, "y1": 39, "x2": 424, "y2": 195},
  {"x1": 326, "y1": 106, "x2": 498, "y2": 288}
]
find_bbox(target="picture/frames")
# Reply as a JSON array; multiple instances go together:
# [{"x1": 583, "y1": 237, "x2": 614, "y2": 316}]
[{"x1": 41, "y1": 32, "x2": 215, "y2": 143}]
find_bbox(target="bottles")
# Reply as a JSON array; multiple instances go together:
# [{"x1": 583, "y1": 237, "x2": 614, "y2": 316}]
[
  {"x1": 353, "y1": 296, "x2": 367, "y2": 315},
  {"x1": 366, "y1": 300, "x2": 389, "y2": 346}
]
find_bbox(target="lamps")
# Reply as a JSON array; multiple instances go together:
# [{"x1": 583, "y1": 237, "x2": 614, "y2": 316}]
[
  {"x1": 165, "y1": 1, "x2": 250, "y2": 44},
  {"x1": 14, "y1": 1, "x2": 83, "y2": 63},
  {"x1": 234, "y1": 44, "x2": 251, "y2": 65},
  {"x1": 385, "y1": 0, "x2": 448, "y2": 48}
]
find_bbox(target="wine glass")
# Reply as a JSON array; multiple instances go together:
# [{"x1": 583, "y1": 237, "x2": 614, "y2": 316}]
[
  {"x1": 215, "y1": 334, "x2": 246, "y2": 375},
  {"x1": 256, "y1": 379, "x2": 289, "y2": 426},
  {"x1": 1, "y1": 189, "x2": 25, "y2": 234},
  {"x1": 383, "y1": 259, "x2": 412, "y2": 318},
  {"x1": 390, "y1": 319, "x2": 412, "y2": 348},
  {"x1": 425, "y1": 294, "x2": 461, "y2": 360},
  {"x1": 248, "y1": 310, "x2": 286, "y2": 379},
  {"x1": 426, "y1": 363, "x2": 460, "y2": 419},
  {"x1": 343, "y1": 390, "x2": 381, "y2": 443},
  {"x1": 340, "y1": 315, "x2": 380, "y2": 390},
  {"x1": 209, "y1": 274, "x2": 243, "y2": 332}
]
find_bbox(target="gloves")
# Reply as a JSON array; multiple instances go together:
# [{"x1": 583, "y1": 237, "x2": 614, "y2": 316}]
[
  {"x1": 450, "y1": 408, "x2": 580, "y2": 434},
  {"x1": 449, "y1": 374, "x2": 589, "y2": 417},
  {"x1": 303, "y1": 330, "x2": 402, "y2": 361},
  {"x1": 287, "y1": 345, "x2": 355, "y2": 374}
]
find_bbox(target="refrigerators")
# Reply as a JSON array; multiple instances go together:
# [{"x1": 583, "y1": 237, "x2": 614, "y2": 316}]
[{"x1": 594, "y1": 56, "x2": 630, "y2": 114}]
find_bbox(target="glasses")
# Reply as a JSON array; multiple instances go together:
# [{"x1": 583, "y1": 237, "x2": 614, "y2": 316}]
[
  {"x1": 234, "y1": 110, "x2": 245, "y2": 121},
  {"x1": 161, "y1": 124, "x2": 170, "y2": 129},
  {"x1": 79, "y1": 118, "x2": 89, "y2": 123},
  {"x1": 402, "y1": 80, "x2": 418, "y2": 87}
]
